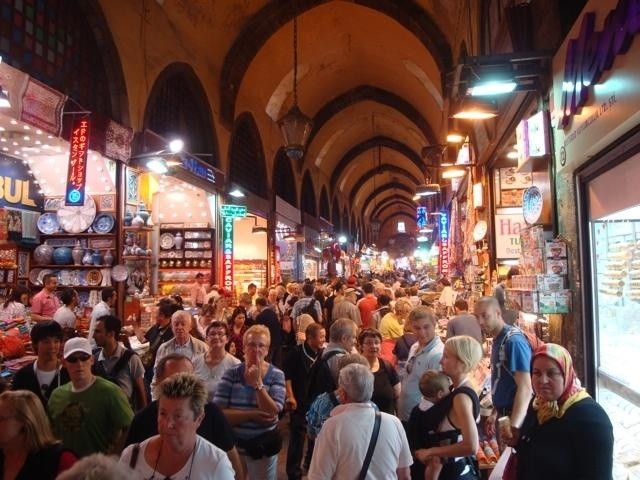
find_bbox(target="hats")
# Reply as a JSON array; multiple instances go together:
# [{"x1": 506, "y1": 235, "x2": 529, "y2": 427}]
[{"x1": 64, "y1": 337, "x2": 92, "y2": 358}]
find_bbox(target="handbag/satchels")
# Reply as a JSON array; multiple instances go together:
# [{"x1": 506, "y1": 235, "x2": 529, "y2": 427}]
[
  {"x1": 239, "y1": 430, "x2": 284, "y2": 460},
  {"x1": 281, "y1": 313, "x2": 293, "y2": 333}
]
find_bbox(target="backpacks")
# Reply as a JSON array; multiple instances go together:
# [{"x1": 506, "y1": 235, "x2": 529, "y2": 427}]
[
  {"x1": 307, "y1": 348, "x2": 344, "y2": 404},
  {"x1": 499, "y1": 325, "x2": 546, "y2": 382},
  {"x1": 300, "y1": 299, "x2": 319, "y2": 322},
  {"x1": 94, "y1": 350, "x2": 137, "y2": 388}
]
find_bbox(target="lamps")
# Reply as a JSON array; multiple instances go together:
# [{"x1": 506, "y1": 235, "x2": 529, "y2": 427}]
[
  {"x1": 245, "y1": 215, "x2": 268, "y2": 234},
  {"x1": 367, "y1": 111, "x2": 384, "y2": 239},
  {"x1": 474, "y1": 182, "x2": 485, "y2": 211},
  {"x1": 275, "y1": 226, "x2": 296, "y2": 240},
  {"x1": 278, "y1": 1, "x2": 314, "y2": 159}
]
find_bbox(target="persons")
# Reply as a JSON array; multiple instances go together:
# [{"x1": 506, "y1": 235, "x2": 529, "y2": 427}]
[
  {"x1": 499, "y1": 342, "x2": 615, "y2": 480},
  {"x1": 1, "y1": 264, "x2": 538, "y2": 479}
]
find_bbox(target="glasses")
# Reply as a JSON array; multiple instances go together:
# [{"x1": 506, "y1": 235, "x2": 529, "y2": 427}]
[
  {"x1": 406, "y1": 357, "x2": 416, "y2": 373},
  {"x1": 66, "y1": 354, "x2": 91, "y2": 363},
  {"x1": 206, "y1": 332, "x2": 228, "y2": 338}
]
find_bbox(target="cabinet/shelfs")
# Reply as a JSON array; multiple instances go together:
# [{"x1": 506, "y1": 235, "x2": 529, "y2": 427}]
[
  {"x1": 123, "y1": 224, "x2": 156, "y2": 320},
  {"x1": 29, "y1": 229, "x2": 115, "y2": 309},
  {"x1": 158, "y1": 228, "x2": 217, "y2": 295}
]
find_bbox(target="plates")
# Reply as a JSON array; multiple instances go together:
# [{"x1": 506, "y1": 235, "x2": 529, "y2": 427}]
[
  {"x1": 519, "y1": 185, "x2": 545, "y2": 225},
  {"x1": 111, "y1": 265, "x2": 129, "y2": 281},
  {"x1": 158, "y1": 231, "x2": 175, "y2": 249},
  {"x1": 472, "y1": 221, "x2": 488, "y2": 242},
  {"x1": 29, "y1": 265, "x2": 102, "y2": 288},
  {"x1": 36, "y1": 192, "x2": 115, "y2": 234}
]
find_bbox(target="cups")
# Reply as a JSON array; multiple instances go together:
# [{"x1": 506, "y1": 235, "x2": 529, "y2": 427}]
[{"x1": 499, "y1": 416, "x2": 513, "y2": 439}]
